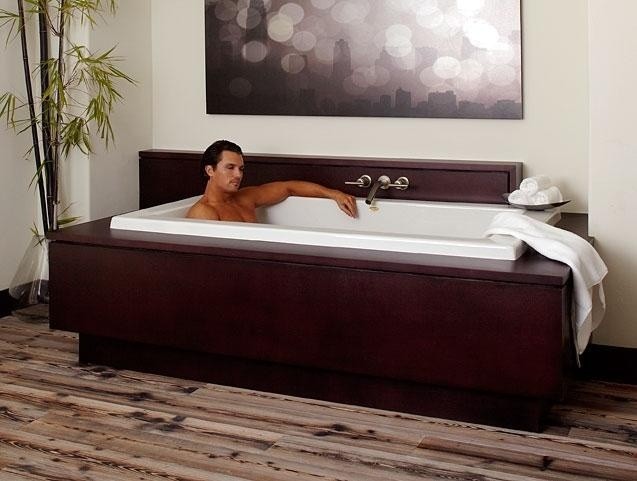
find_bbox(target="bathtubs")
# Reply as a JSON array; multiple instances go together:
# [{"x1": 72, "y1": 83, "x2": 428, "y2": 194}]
[{"x1": 110, "y1": 195, "x2": 562, "y2": 262}]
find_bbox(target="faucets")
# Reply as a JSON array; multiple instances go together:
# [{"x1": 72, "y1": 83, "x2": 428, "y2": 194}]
[{"x1": 365, "y1": 176, "x2": 390, "y2": 205}]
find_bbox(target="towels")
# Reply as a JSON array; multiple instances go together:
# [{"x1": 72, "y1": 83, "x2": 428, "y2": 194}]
[
  {"x1": 509, "y1": 174, "x2": 562, "y2": 205},
  {"x1": 483, "y1": 211, "x2": 610, "y2": 356}
]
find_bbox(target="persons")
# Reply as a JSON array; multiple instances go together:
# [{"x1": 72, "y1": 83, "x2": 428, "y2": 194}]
[{"x1": 181, "y1": 139, "x2": 360, "y2": 232}]
